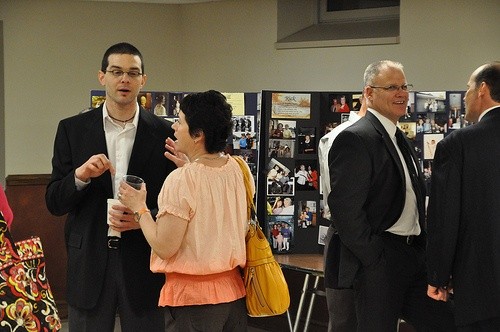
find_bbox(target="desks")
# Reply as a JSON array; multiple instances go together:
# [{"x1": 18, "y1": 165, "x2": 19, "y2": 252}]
[{"x1": 274, "y1": 255, "x2": 325, "y2": 332}]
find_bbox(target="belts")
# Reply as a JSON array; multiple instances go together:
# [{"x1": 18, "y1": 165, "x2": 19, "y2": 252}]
[
  {"x1": 108, "y1": 238, "x2": 120, "y2": 249},
  {"x1": 385, "y1": 232, "x2": 419, "y2": 246}
]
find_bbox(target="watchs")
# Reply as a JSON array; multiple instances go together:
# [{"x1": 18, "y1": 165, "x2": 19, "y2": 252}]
[{"x1": 133, "y1": 208, "x2": 150, "y2": 223}]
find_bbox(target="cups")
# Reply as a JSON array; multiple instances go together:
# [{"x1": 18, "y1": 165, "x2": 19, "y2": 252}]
[
  {"x1": 106, "y1": 198, "x2": 125, "y2": 224},
  {"x1": 117, "y1": 175, "x2": 144, "y2": 206}
]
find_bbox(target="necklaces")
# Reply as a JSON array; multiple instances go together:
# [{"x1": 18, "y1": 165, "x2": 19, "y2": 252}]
[{"x1": 109, "y1": 111, "x2": 135, "y2": 125}]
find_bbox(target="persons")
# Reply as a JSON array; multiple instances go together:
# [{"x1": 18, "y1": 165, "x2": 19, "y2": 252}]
[
  {"x1": 331, "y1": 96, "x2": 349, "y2": 124},
  {"x1": 172, "y1": 96, "x2": 179, "y2": 115},
  {"x1": 45, "y1": 43, "x2": 176, "y2": 332},
  {"x1": 320, "y1": 92, "x2": 367, "y2": 225},
  {"x1": 117, "y1": 89, "x2": 255, "y2": 332},
  {"x1": 401, "y1": 98, "x2": 467, "y2": 179},
  {"x1": 235, "y1": 118, "x2": 251, "y2": 132},
  {"x1": 267, "y1": 165, "x2": 317, "y2": 251},
  {"x1": 239, "y1": 133, "x2": 253, "y2": 149},
  {"x1": 272, "y1": 123, "x2": 292, "y2": 138},
  {"x1": 271, "y1": 146, "x2": 290, "y2": 158},
  {"x1": 153, "y1": 94, "x2": 166, "y2": 116},
  {"x1": 424, "y1": 62, "x2": 500, "y2": 332},
  {"x1": 301, "y1": 134, "x2": 315, "y2": 154},
  {"x1": 325, "y1": 61, "x2": 427, "y2": 332},
  {"x1": 0, "y1": 186, "x2": 14, "y2": 228},
  {"x1": 140, "y1": 96, "x2": 148, "y2": 110}
]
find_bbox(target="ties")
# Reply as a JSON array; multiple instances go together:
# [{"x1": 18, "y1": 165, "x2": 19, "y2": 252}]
[{"x1": 395, "y1": 127, "x2": 425, "y2": 238}]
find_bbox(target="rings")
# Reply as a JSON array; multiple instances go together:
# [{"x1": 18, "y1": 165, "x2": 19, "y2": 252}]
[{"x1": 118, "y1": 194, "x2": 122, "y2": 199}]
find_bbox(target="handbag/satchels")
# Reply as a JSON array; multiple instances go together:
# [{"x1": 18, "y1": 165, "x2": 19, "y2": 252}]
[{"x1": 233, "y1": 156, "x2": 291, "y2": 317}]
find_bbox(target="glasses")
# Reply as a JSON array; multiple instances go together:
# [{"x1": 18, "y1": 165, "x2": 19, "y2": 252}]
[
  {"x1": 367, "y1": 84, "x2": 413, "y2": 91},
  {"x1": 105, "y1": 70, "x2": 143, "y2": 77}
]
